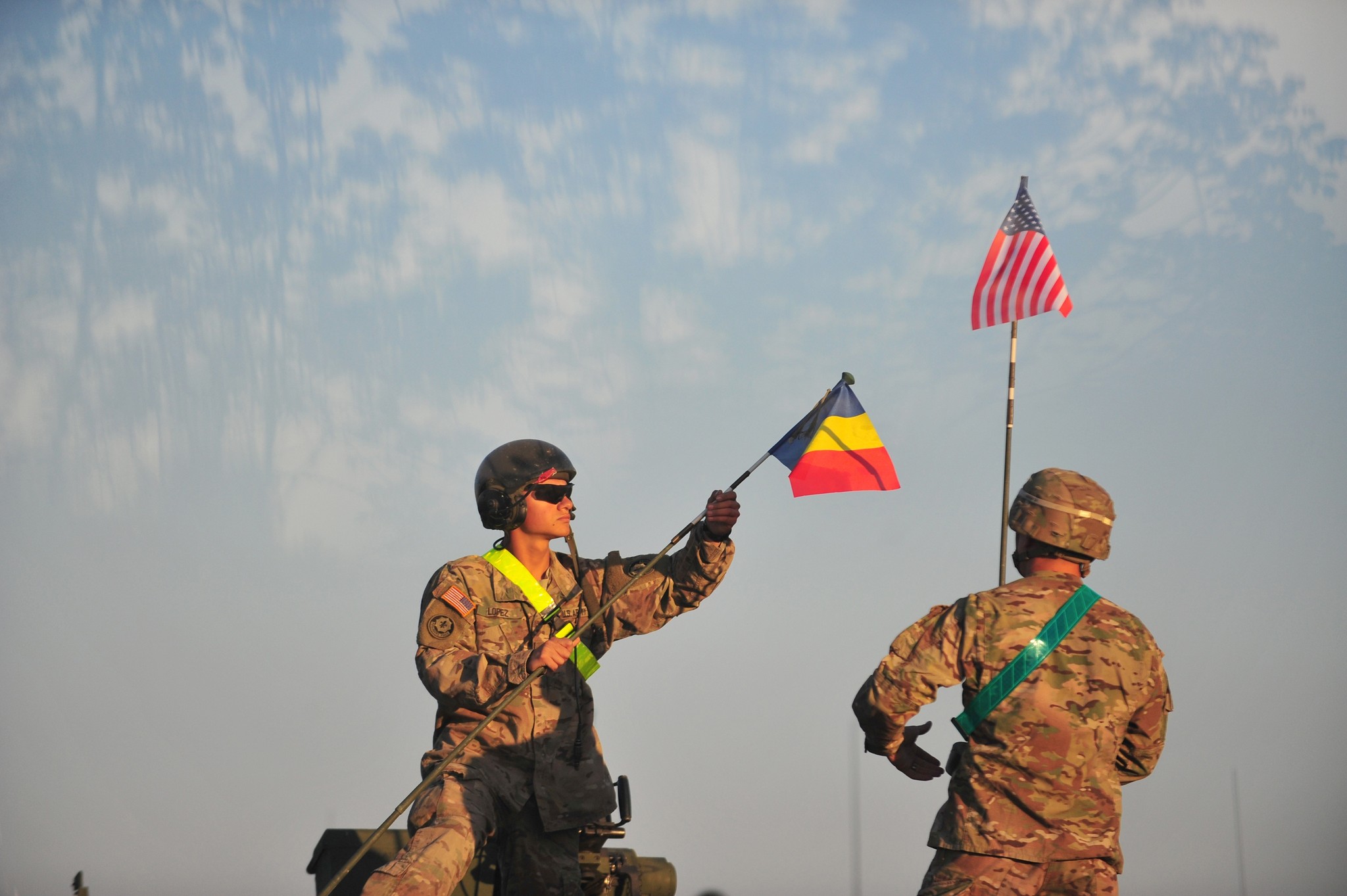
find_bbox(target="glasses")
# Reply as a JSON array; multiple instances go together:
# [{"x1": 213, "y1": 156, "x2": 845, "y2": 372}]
[{"x1": 525, "y1": 483, "x2": 575, "y2": 505}]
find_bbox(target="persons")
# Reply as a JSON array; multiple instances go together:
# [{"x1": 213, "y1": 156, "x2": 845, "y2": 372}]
[
  {"x1": 355, "y1": 440, "x2": 739, "y2": 896},
  {"x1": 850, "y1": 466, "x2": 1173, "y2": 896}
]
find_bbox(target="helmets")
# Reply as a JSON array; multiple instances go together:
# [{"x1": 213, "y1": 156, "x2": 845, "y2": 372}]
[
  {"x1": 1009, "y1": 468, "x2": 1116, "y2": 561},
  {"x1": 475, "y1": 439, "x2": 577, "y2": 531}
]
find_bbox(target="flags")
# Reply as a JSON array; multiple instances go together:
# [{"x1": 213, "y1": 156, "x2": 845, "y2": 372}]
[
  {"x1": 770, "y1": 380, "x2": 901, "y2": 498},
  {"x1": 970, "y1": 185, "x2": 1073, "y2": 329}
]
